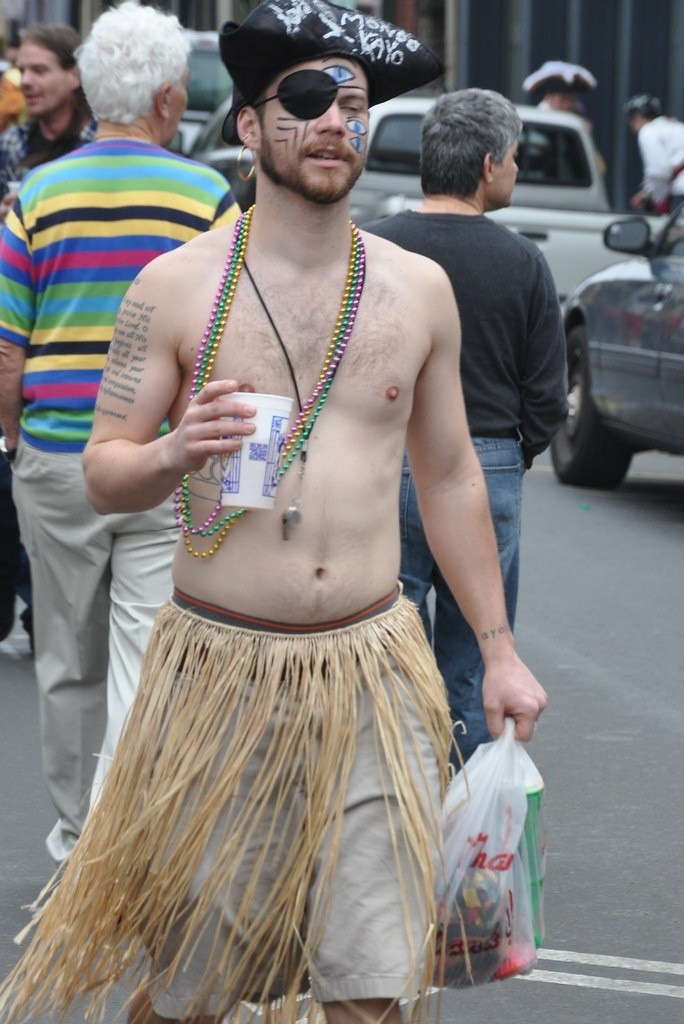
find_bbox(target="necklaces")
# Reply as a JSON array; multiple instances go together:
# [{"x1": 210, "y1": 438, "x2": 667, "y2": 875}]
[{"x1": 174, "y1": 204, "x2": 367, "y2": 560}]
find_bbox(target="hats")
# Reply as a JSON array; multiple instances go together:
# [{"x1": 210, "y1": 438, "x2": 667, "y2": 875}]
[{"x1": 219, "y1": 1, "x2": 447, "y2": 146}]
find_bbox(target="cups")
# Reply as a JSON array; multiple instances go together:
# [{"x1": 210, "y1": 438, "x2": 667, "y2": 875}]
[{"x1": 217, "y1": 391, "x2": 296, "y2": 506}]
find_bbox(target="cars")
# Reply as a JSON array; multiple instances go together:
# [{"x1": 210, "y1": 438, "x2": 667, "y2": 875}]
[{"x1": 551, "y1": 199, "x2": 683, "y2": 492}]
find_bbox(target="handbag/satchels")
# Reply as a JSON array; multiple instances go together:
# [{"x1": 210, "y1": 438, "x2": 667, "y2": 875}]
[{"x1": 421, "y1": 714, "x2": 548, "y2": 990}]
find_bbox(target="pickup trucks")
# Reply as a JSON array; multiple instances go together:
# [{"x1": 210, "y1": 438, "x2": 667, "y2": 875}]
[{"x1": 347, "y1": 98, "x2": 673, "y2": 308}]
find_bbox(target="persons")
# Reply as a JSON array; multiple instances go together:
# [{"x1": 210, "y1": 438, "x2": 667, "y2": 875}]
[
  {"x1": 0, "y1": 0, "x2": 550, "y2": 1023},
  {"x1": 1, "y1": 23, "x2": 101, "y2": 660},
  {"x1": 0, "y1": 32, "x2": 32, "y2": 135},
  {"x1": 1, "y1": 0, "x2": 243, "y2": 886},
  {"x1": 352, "y1": 84, "x2": 572, "y2": 778},
  {"x1": 624, "y1": 92, "x2": 684, "y2": 216},
  {"x1": 535, "y1": 85, "x2": 587, "y2": 118}
]
